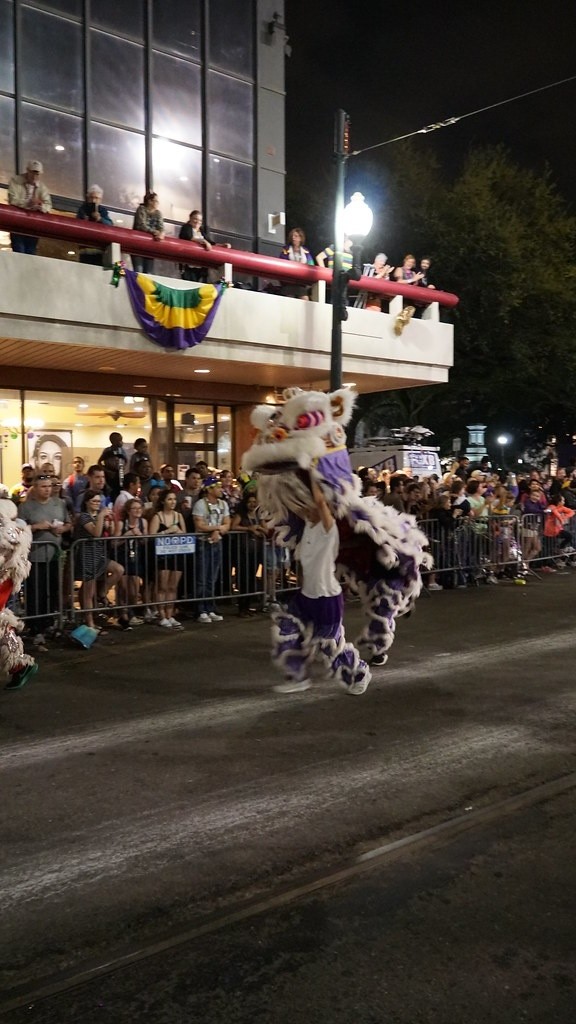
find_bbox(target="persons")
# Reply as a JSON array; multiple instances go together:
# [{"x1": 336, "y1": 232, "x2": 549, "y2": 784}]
[
  {"x1": 8, "y1": 160, "x2": 52, "y2": 255},
  {"x1": 33, "y1": 434, "x2": 73, "y2": 480},
  {"x1": 130, "y1": 193, "x2": 165, "y2": 274},
  {"x1": 76, "y1": 185, "x2": 114, "y2": 267},
  {"x1": 12, "y1": 432, "x2": 576, "y2": 651},
  {"x1": 178, "y1": 210, "x2": 231, "y2": 283},
  {"x1": 395, "y1": 254, "x2": 435, "y2": 318},
  {"x1": 271, "y1": 459, "x2": 373, "y2": 695},
  {"x1": 279, "y1": 228, "x2": 314, "y2": 300},
  {"x1": 364, "y1": 253, "x2": 394, "y2": 312},
  {"x1": 316, "y1": 237, "x2": 354, "y2": 306}
]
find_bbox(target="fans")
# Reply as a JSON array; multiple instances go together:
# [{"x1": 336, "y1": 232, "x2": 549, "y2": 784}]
[{"x1": 73, "y1": 409, "x2": 147, "y2": 421}]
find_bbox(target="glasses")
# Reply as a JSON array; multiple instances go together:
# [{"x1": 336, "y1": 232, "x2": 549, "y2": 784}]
[
  {"x1": 89, "y1": 498, "x2": 103, "y2": 504},
  {"x1": 37, "y1": 475, "x2": 50, "y2": 480},
  {"x1": 52, "y1": 483, "x2": 64, "y2": 487},
  {"x1": 129, "y1": 506, "x2": 143, "y2": 512}
]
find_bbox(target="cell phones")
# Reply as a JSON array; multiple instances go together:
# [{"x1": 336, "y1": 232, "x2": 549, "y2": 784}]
[
  {"x1": 87, "y1": 203, "x2": 96, "y2": 216},
  {"x1": 208, "y1": 535, "x2": 223, "y2": 543}
]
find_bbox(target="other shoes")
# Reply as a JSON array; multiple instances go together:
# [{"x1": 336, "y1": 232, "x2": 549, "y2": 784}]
[
  {"x1": 440, "y1": 546, "x2": 576, "y2": 588},
  {"x1": 370, "y1": 653, "x2": 388, "y2": 666},
  {"x1": 428, "y1": 584, "x2": 443, "y2": 590},
  {"x1": 271, "y1": 677, "x2": 313, "y2": 693},
  {"x1": 345, "y1": 590, "x2": 361, "y2": 602},
  {"x1": 8, "y1": 662, "x2": 38, "y2": 690},
  {"x1": 404, "y1": 596, "x2": 415, "y2": 618},
  {"x1": 347, "y1": 671, "x2": 372, "y2": 695},
  {"x1": 8, "y1": 569, "x2": 308, "y2": 655}
]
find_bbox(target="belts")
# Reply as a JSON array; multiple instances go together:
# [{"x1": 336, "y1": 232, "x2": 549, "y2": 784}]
[{"x1": 474, "y1": 520, "x2": 487, "y2": 525}]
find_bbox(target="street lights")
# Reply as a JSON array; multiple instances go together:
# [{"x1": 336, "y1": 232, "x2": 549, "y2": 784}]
[
  {"x1": 498, "y1": 435, "x2": 508, "y2": 469},
  {"x1": 328, "y1": 107, "x2": 373, "y2": 394}
]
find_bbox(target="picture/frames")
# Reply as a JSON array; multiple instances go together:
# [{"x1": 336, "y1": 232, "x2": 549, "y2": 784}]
[{"x1": 27, "y1": 429, "x2": 73, "y2": 479}]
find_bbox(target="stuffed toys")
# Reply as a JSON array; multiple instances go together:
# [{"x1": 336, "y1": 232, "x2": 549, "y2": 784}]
[
  {"x1": 0, "y1": 499, "x2": 38, "y2": 690},
  {"x1": 241, "y1": 386, "x2": 434, "y2": 666}
]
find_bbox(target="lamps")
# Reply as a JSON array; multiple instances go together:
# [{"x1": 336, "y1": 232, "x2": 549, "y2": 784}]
[{"x1": 2, "y1": 417, "x2": 44, "y2": 434}]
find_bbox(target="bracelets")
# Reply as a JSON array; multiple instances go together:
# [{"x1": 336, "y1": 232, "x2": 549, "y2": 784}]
[{"x1": 409, "y1": 279, "x2": 414, "y2": 283}]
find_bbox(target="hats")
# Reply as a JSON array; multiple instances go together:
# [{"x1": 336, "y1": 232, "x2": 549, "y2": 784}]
[
  {"x1": 28, "y1": 160, "x2": 43, "y2": 174},
  {"x1": 443, "y1": 472, "x2": 457, "y2": 482},
  {"x1": 203, "y1": 476, "x2": 222, "y2": 488},
  {"x1": 21, "y1": 463, "x2": 33, "y2": 473},
  {"x1": 103, "y1": 451, "x2": 125, "y2": 461},
  {"x1": 471, "y1": 470, "x2": 487, "y2": 479}
]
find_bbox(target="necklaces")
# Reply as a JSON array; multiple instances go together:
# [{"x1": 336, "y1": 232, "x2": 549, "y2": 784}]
[{"x1": 163, "y1": 511, "x2": 175, "y2": 523}]
